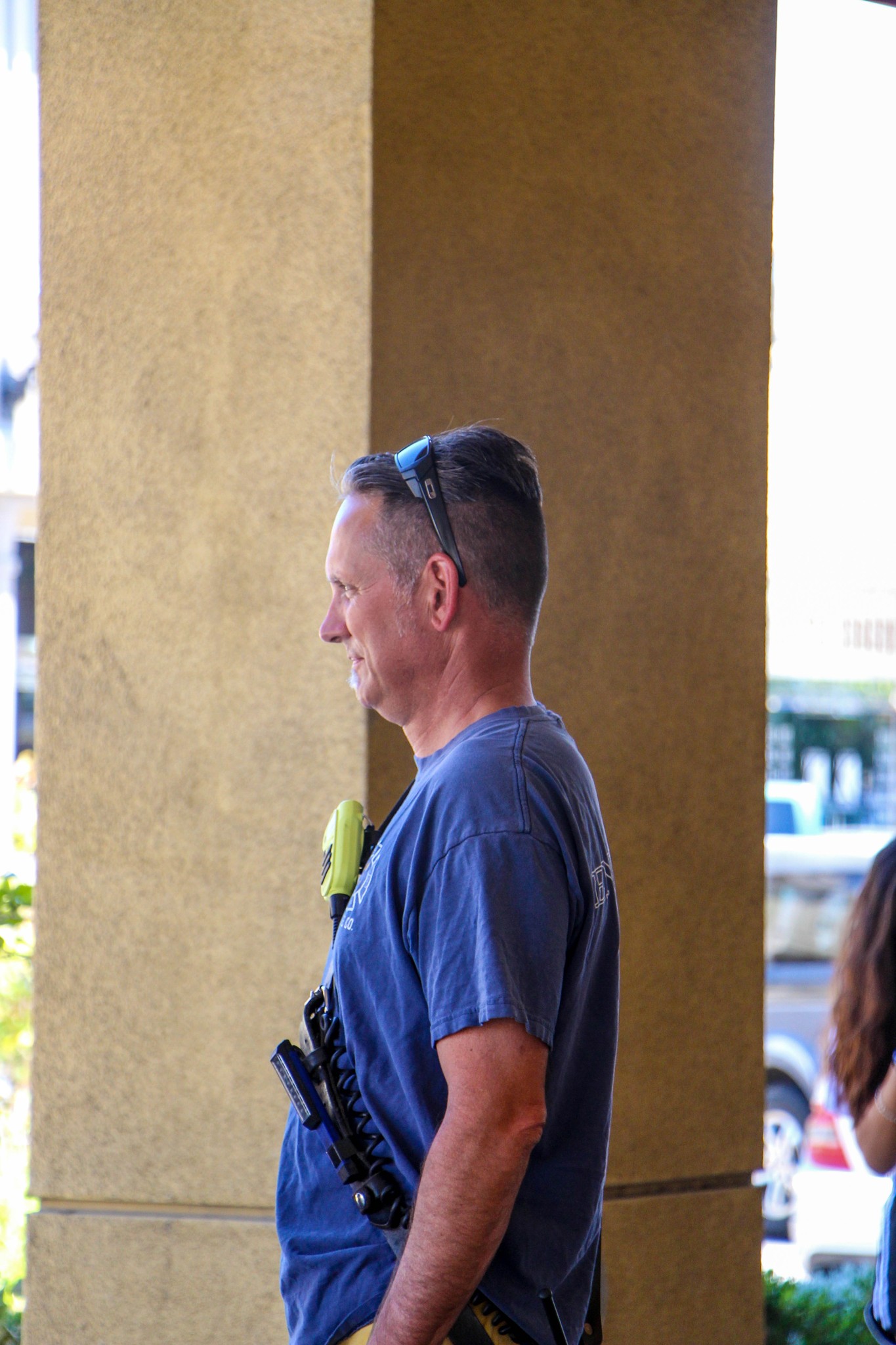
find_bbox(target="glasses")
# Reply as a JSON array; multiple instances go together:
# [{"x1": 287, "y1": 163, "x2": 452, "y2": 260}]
[{"x1": 394, "y1": 435, "x2": 466, "y2": 588}]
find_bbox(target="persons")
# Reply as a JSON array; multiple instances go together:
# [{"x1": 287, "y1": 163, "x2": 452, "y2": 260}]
[
  {"x1": 274, "y1": 425, "x2": 621, "y2": 1345},
  {"x1": 831, "y1": 836, "x2": 896, "y2": 1344}
]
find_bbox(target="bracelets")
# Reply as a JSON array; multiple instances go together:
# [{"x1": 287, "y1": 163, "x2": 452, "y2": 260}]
[{"x1": 875, "y1": 1085, "x2": 896, "y2": 1123}]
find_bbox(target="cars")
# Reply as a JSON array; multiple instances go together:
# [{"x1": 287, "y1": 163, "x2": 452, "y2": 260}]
[
  {"x1": 791, "y1": 1066, "x2": 896, "y2": 1281},
  {"x1": 761, "y1": 1027, "x2": 822, "y2": 1235}
]
